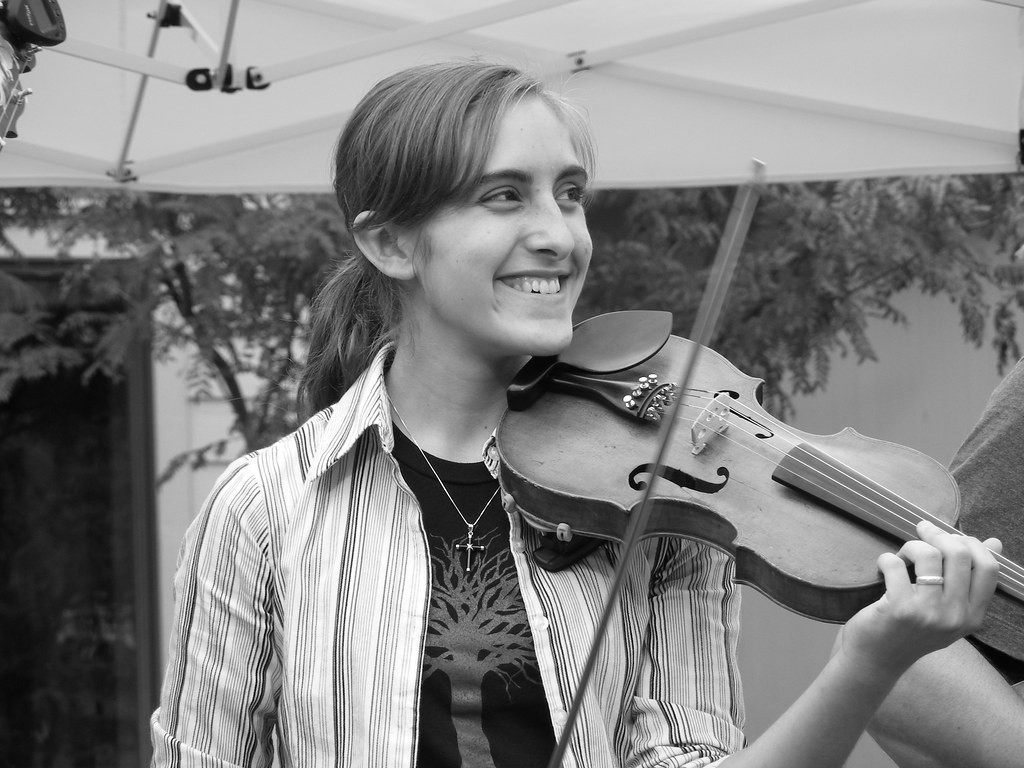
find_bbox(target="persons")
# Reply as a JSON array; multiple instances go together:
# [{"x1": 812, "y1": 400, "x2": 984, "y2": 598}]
[
  {"x1": 865, "y1": 358, "x2": 1024, "y2": 768},
  {"x1": 148, "y1": 58, "x2": 1004, "y2": 768}
]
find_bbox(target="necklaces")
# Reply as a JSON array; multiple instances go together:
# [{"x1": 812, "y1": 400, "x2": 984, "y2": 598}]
[{"x1": 387, "y1": 395, "x2": 501, "y2": 571}]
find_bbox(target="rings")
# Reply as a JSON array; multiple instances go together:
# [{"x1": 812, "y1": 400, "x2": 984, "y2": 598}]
[{"x1": 916, "y1": 576, "x2": 944, "y2": 585}]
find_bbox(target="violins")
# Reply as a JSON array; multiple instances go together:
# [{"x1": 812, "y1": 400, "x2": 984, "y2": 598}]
[{"x1": 495, "y1": 311, "x2": 1024, "y2": 625}]
